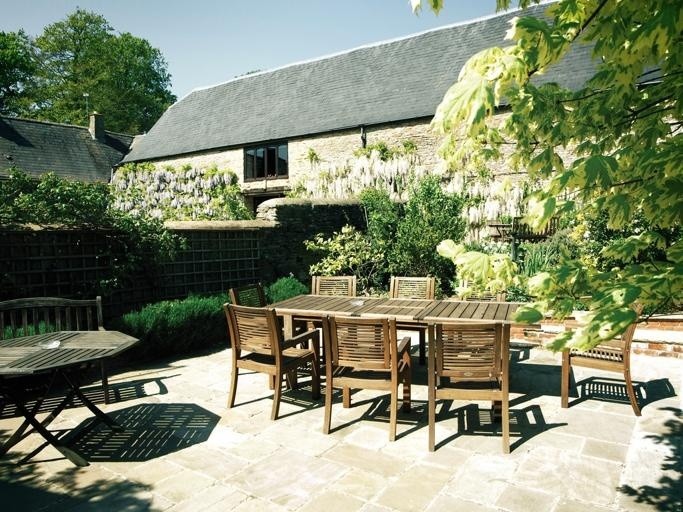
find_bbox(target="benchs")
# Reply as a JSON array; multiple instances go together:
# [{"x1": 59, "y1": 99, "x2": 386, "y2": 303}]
[{"x1": 0, "y1": 295, "x2": 110, "y2": 405}]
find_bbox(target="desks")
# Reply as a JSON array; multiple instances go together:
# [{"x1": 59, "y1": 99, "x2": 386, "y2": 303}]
[{"x1": 0, "y1": 329, "x2": 141, "y2": 468}]
